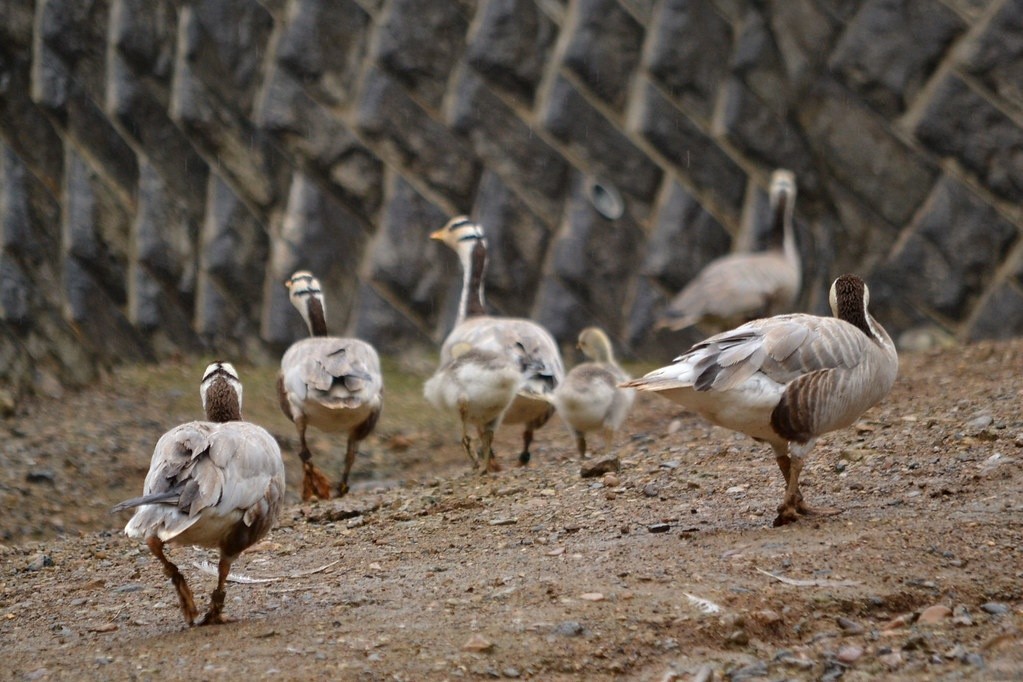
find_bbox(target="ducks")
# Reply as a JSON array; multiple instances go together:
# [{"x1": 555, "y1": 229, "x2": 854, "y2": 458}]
[
  {"x1": 649, "y1": 169, "x2": 803, "y2": 346},
  {"x1": 420, "y1": 216, "x2": 565, "y2": 476},
  {"x1": 613, "y1": 272, "x2": 901, "y2": 527},
  {"x1": 275, "y1": 270, "x2": 383, "y2": 500},
  {"x1": 547, "y1": 327, "x2": 636, "y2": 457},
  {"x1": 112, "y1": 360, "x2": 288, "y2": 629}
]
